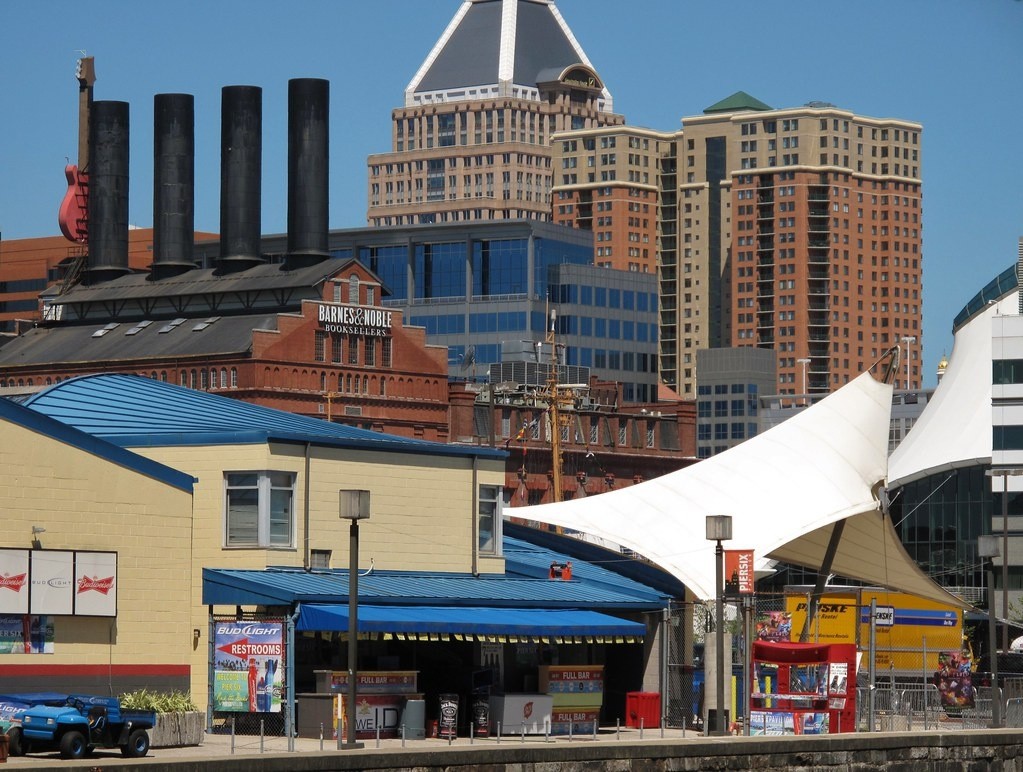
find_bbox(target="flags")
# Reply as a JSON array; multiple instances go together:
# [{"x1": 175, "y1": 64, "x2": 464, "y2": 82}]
[
  {"x1": 516, "y1": 428, "x2": 524, "y2": 440},
  {"x1": 523, "y1": 440, "x2": 527, "y2": 456},
  {"x1": 523, "y1": 417, "x2": 528, "y2": 430}
]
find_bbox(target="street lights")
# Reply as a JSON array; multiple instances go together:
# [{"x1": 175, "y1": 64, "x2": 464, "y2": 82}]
[
  {"x1": 900, "y1": 336, "x2": 916, "y2": 391},
  {"x1": 977, "y1": 533, "x2": 1006, "y2": 730},
  {"x1": 705, "y1": 515, "x2": 733, "y2": 735},
  {"x1": 984, "y1": 468, "x2": 1022, "y2": 654},
  {"x1": 797, "y1": 357, "x2": 811, "y2": 406},
  {"x1": 339, "y1": 489, "x2": 371, "y2": 750}
]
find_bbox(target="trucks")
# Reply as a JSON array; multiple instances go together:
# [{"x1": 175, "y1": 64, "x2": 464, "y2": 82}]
[{"x1": 5, "y1": 694, "x2": 156, "y2": 760}]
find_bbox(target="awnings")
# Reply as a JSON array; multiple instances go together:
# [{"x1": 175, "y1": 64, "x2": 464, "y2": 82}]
[{"x1": 296, "y1": 601, "x2": 648, "y2": 647}]
